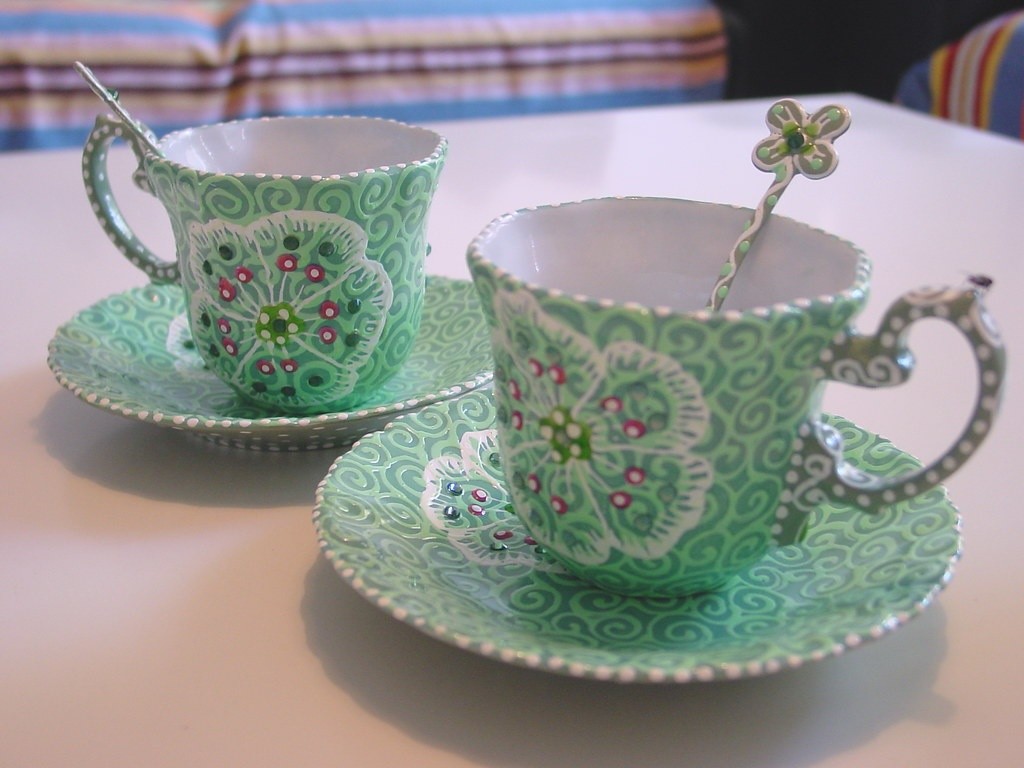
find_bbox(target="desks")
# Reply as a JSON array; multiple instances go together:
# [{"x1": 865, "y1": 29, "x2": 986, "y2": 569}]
[{"x1": 0, "y1": 93, "x2": 1024, "y2": 768}]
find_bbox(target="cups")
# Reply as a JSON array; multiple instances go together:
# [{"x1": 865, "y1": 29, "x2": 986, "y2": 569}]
[
  {"x1": 466, "y1": 195, "x2": 1007, "y2": 598},
  {"x1": 80, "y1": 110, "x2": 448, "y2": 414}
]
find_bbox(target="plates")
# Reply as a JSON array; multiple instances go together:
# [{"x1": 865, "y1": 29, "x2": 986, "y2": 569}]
[
  {"x1": 315, "y1": 380, "x2": 964, "y2": 684},
  {"x1": 49, "y1": 273, "x2": 494, "y2": 450}
]
